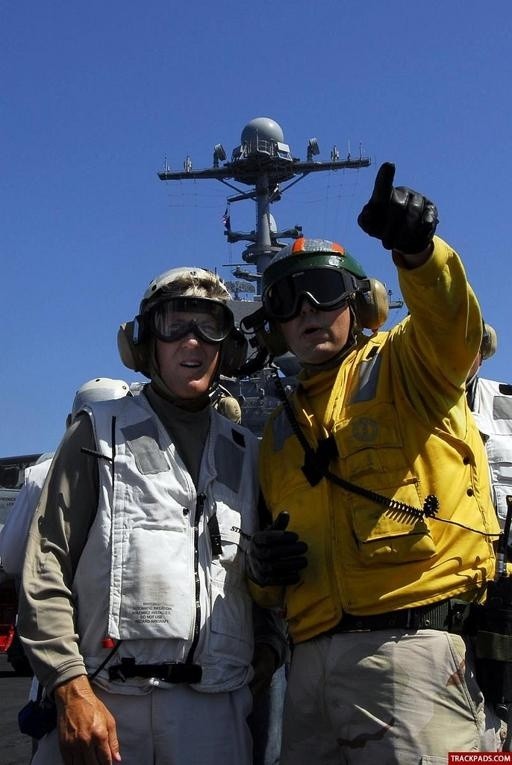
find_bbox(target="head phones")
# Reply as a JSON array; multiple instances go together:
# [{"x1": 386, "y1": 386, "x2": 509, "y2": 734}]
[
  {"x1": 252, "y1": 278, "x2": 390, "y2": 358},
  {"x1": 116, "y1": 321, "x2": 249, "y2": 378}
]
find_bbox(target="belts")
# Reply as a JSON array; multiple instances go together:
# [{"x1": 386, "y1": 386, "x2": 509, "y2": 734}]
[{"x1": 130, "y1": 664, "x2": 203, "y2": 683}]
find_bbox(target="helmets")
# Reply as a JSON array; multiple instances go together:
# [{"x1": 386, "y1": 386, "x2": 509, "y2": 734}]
[
  {"x1": 260, "y1": 237, "x2": 369, "y2": 316},
  {"x1": 139, "y1": 266, "x2": 235, "y2": 322},
  {"x1": 66, "y1": 377, "x2": 130, "y2": 429}
]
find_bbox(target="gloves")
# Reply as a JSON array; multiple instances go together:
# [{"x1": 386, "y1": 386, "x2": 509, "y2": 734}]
[
  {"x1": 240, "y1": 510, "x2": 308, "y2": 587},
  {"x1": 357, "y1": 161, "x2": 439, "y2": 255}
]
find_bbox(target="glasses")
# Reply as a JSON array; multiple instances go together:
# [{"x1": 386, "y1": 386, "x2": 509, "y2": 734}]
[
  {"x1": 262, "y1": 266, "x2": 369, "y2": 323},
  {"x1": 141, "y1": 296, "x2": 235, "y2": 345}
]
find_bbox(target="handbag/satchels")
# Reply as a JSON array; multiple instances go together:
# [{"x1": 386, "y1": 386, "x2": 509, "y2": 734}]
[{"x1": 475, "y1": 572, "x2": 512, "y2": 721}]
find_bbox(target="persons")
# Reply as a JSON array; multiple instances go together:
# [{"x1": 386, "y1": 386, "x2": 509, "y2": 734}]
[
  {"x1": 13, "y1": 266, "x2": 287, "y2": 765},
  {"x1": 240, "y1": 158, "x2": 511, "y2": 765},
  {"x1": 462, "y1": 316, "x2": 511, "y2": 761},
  {"x1": 1, "y1": 447, "x2": 59, "y2": 760}
]
